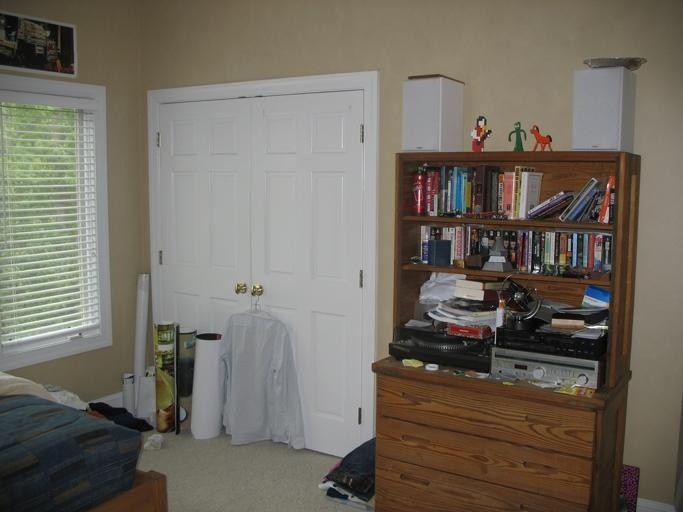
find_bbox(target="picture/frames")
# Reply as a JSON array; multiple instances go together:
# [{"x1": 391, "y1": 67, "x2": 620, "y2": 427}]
[{"x1": 0, "y1": 10, "x2": 77, "y2": 80}]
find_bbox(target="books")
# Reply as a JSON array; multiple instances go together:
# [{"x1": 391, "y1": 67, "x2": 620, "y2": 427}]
[
  {"x1": 425, "y1": 278, "x2": 512, "y2": 341},
  {"x1": 408, "y1": 163, "x2": 614, "y2": 282},
  {"x1": 550, "y1": 309, "x2": 609, "y2": 328}
]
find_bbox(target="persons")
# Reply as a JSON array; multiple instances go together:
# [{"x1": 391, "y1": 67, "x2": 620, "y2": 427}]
[
  {"x1": 470, "y1": 115, "x2": 493, "y2": 152},
  {"x1": 507, "y1": 121, "x2": 527, "y2": 152}
]
